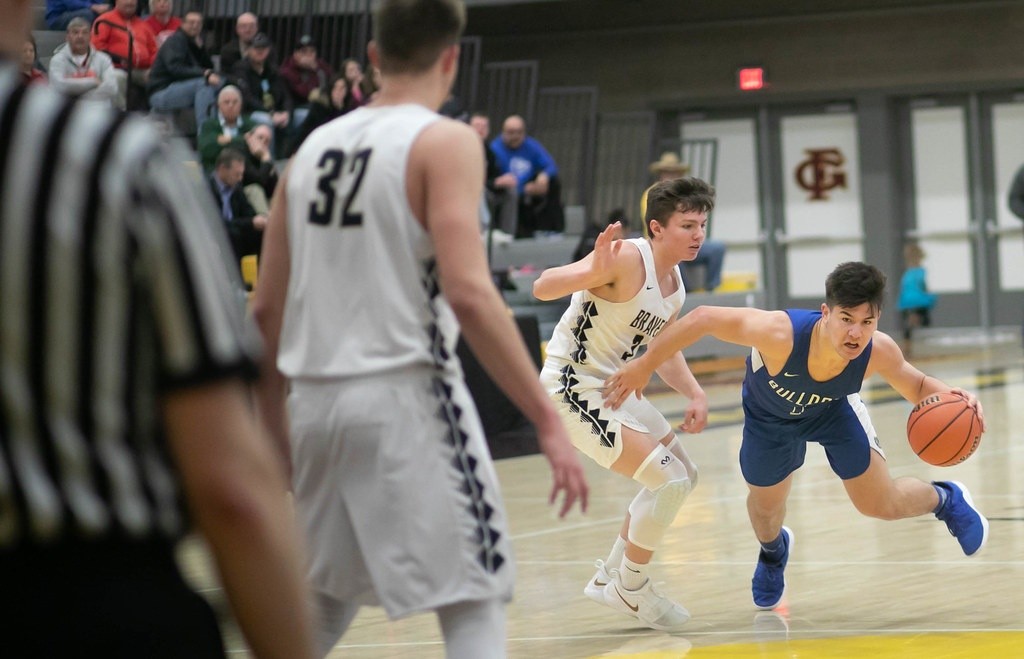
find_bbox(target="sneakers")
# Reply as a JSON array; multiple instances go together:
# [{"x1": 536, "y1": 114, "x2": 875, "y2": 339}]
[
  {"x1": 751, "y1": 526, "x2": 794, "y2": 610},
  {"x1": 585, "y1": 560, "x2": 615, "y2": 606},
  {"x1": 604, "y1": 567, "x2": 690, "y2": 630},
  {"x1": 931, "y1": 480, "x2": 988, "y2": 558}
]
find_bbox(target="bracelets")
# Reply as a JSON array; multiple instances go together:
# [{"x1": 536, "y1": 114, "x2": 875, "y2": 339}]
[{"x1": 205, "y1": 71, "x2": 213, "y2": 84}]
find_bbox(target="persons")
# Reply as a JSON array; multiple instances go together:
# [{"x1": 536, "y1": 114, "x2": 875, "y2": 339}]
[
  {"x1": 639, "y1": 154, "x2": 725, "y2": 294},
  {"x1": 572, "y1": 205, "x2": 629, "y2": 264},
  {"x1": 253, "y1": 0, "x2": 589, "y2": 659},
  {"x1": 533, "y1": 175, "x2": 714, "y2": 633},
  {"x1": 466, "y1": 110, "x2": 519, "y2": 240},
  {"x1": 0, "y1": 0, "x2": 325, "y2": 659},
  {"x1": 895, "y1": 243, "x2": 934, "y2": 356},
  {"x1": 16, "y1": 35, "x2": 44, "y2": 83},
  {"x1": 489, "y1": 111, "x2": 564, "y2": 241},
  {"x1": 601, "y1": 261, "x2": 988, "y2": 609},
  {"x1": 41, "y1": 0, "x2": 382, "y2": 295}
]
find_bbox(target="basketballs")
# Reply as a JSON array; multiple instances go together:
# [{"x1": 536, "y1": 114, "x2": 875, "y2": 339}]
[{"x1": 906, "y1": 391, "x2": 981, "y2": 467}]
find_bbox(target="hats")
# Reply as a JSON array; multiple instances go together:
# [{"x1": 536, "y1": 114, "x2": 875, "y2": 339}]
[
  {"x1": 648, "y1": 151, "x2": 692, "y2": 175},
  {"x1": 252, "y1": 31, "x2": 271, "y2": 48},
  {"x1": 293, "y1": 37, "x2": 314, "y2": 51}
]
